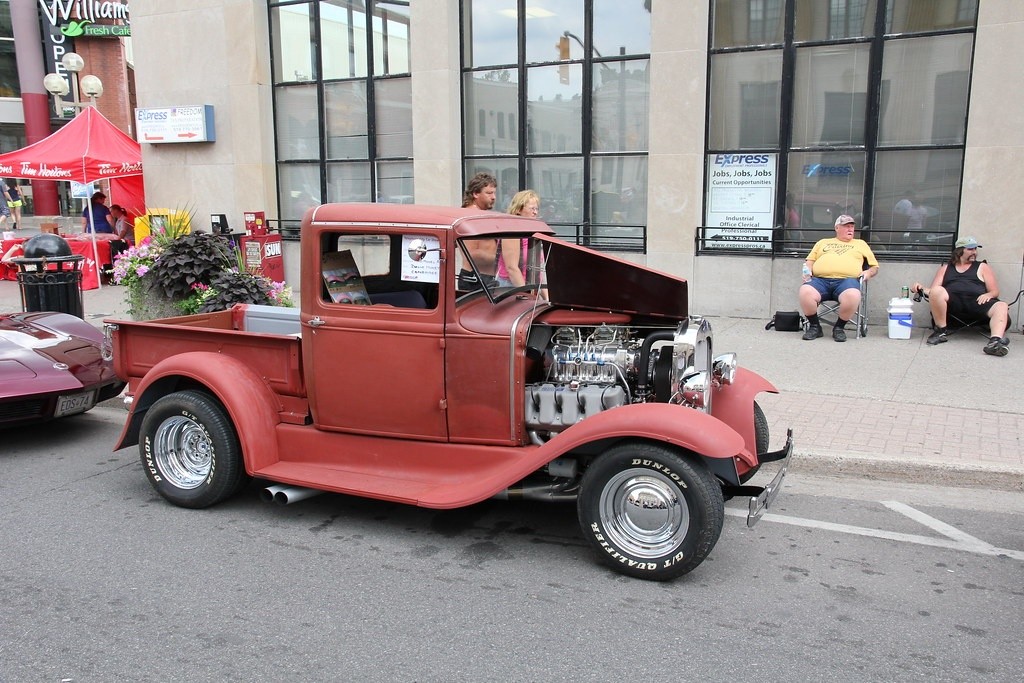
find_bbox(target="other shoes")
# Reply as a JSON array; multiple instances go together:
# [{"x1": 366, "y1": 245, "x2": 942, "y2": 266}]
[{"x1": 13, "y1": 222, "x2": 16, "y2": 229}]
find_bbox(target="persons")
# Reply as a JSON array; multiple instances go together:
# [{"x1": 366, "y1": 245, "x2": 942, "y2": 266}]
[
  {"x1": 0, "y1": 177, "x2": 27, "y2": 230},
  {"x1": 894, "y1": 190, "x2": 927, "y2": 242},
  {"x1": 786, "y1": 205, "x2": 805, "y2": 248},
  {"x1": 910, "y1": 237, "x2": 1009, "y2": 356},
  {"x1": 110, "y1": 205, "x2": 134, "y2": 247},
  {"x1": 82, "y1": 192, "x2": 114, "y2": 233},
  {"x1": 456, "y1": 172, "x2": 498, "y2": 292},
  {"x1": 799, "y1": 215, "x2": 880, "y2": 341},
  {"x1": 497, "y1": 189, "x2": 549, "y2": 301}
]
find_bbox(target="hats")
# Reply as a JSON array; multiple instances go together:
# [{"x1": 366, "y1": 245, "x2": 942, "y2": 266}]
[
  {"x1": 835, "y1": 215, "x2": 856, "y2": 225},
  {"x1": 955, "y1": 236, "x2": 982, "y2": 248}
]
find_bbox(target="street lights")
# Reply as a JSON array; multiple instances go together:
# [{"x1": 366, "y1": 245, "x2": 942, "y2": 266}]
[{"x1": 43, "y1": 52, "x2": 104, "y2": 119}]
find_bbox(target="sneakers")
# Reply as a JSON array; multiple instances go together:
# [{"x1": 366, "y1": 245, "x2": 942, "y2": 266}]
[
  {"x1": 983, "y1": 336, "x2": 1011, "y2": 356},
  {"x1": 926, "y1": 326, "x2": 948, "y2": 345},
  {"x1": 803, "y1": 324, "x2": 823, "y2": 340},
  {"x1": 832, "y1": 327, "x2": 847, "y2": 342}
]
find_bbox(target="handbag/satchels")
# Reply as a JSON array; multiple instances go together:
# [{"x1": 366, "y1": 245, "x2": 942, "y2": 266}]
[{"x1": 774, "y1": 311, "x2": 799, "y2": 332}]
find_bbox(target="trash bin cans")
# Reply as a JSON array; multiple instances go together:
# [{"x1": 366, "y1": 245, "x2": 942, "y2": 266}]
[{"x1": 11, "y1": 234, "x2": 86, "y2": 320}]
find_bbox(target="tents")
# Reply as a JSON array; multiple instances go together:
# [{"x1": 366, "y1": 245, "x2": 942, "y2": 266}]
[{"x1": 0, "y1": 105, "x2": 145, "y2": 289}]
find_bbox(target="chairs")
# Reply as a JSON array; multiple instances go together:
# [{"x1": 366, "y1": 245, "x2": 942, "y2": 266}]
[
  {"x1": 102, "y1": 239, "x2": 130, "y2": 286},
  {"x1": 803, "y1": 274, "x2": 867, "y2": 340},
  {"x1": 377, "y1": 290, "x2": 426, "y2": 309}
]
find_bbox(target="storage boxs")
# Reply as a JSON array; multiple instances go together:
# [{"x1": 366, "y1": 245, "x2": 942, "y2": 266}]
[
  {"x1": 40, "y1": 223, "x2": 59, "y2": 235},
  {"x1": 888, "y1": 314, "x2": 913, "y2": 339}
]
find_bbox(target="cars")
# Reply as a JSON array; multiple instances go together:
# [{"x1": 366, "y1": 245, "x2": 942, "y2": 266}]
[{"x1": 100, "y1": 199, "x2": 795, "y2": 584}]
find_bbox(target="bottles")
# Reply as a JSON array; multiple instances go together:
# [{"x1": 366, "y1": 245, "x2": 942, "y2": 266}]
[{"x1": 803, "y1": 263, "x2": 811, "y2": 283}]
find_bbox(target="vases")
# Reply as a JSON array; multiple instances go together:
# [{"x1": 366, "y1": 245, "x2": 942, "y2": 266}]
[{"x1": 142, "y1": 272, "x2": 190, "y2": 320}]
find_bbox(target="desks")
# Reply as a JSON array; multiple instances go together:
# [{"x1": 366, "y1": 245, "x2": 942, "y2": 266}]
[{"x1": 0, "y1": 235, "x2": 134, "y2": 291}]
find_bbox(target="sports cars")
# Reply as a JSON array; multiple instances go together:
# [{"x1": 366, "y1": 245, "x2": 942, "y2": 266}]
[{"x1": 0, "y1": 309, "x2": 128, "y2": 427}]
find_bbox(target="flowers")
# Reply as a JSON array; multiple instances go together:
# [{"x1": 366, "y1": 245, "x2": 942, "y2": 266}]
[
  {"x1": 111, "y1": 225, "x2": 169, "y2": 285},
  {"x1": 192, "y1": 239, "x2": 293, "y2": 308}
]
